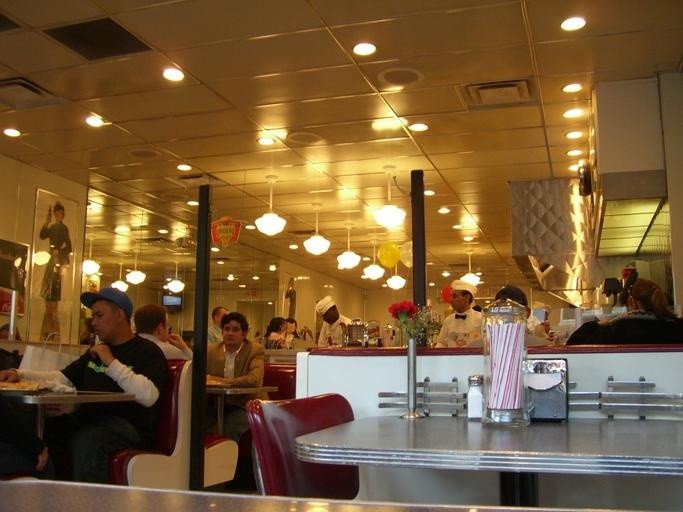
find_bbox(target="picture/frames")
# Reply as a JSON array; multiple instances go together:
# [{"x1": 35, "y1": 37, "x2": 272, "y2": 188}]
[{"x1": 28, "y1": 188, "x2": 79, "y2": 344}]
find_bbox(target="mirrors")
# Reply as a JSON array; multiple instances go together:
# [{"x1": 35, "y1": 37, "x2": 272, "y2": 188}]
[{"x1": 78, "y1": 172, "x2": 276, "y2": 350}]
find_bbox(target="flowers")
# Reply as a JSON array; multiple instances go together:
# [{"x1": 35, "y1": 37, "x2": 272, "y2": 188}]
[{"x1": 389, "y1": 298, "x2": 419, "y2": 326}]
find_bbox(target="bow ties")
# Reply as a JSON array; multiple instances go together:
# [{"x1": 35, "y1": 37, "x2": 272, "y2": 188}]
[{"x1": 454, "y1": 313, "x2": 468, "y2": 320}]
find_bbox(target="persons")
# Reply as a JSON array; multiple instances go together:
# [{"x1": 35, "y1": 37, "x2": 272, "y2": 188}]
[{"x1": 40, "y1": 200, "x2": 73, "y2": 334}]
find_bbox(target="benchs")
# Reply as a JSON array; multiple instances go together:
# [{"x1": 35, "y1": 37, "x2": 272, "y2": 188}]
[{"x1": 1, "y1": 360, "x2": 297, "y2": 492}]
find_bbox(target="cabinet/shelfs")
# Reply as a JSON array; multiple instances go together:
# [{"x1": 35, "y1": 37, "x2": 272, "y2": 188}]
[{"x1": 586, "y1": 80, "x2": 666, "y2": 260}]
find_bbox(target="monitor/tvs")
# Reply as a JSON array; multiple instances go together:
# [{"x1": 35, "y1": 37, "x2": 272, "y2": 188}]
[{"x1": 162, "y1": 295, "x2": 183, "y2": 306}]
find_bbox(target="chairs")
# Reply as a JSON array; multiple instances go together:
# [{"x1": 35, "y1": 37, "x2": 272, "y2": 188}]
[{"x1": 243, "y1": 390, "x2": 363, "y2": 502}]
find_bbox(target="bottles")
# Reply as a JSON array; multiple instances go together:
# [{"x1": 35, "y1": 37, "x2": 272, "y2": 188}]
[
  {"x1": 347, "y1": 318, "x2": 364, "y2": 343},
  {"x1": 382, "y1": 324, "x2": 394, "y2": 347},
  {"x1": 479, "y1": 294, "x2": 531, "y2": 428},
  {"x1": 465, "y1": 375, "x2": 483, "y2": 419}
]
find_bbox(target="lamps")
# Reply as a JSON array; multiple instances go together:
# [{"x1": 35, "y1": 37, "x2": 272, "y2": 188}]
[
  {"x1": 459, "y1": 250, "x2": 481, "y2": 287},
  {"x1": 253, "y1": 176, "x2": 406, "y2": 291},
  {"x1": 84, "y1": 237, "x2": 186, "y2": 296}
]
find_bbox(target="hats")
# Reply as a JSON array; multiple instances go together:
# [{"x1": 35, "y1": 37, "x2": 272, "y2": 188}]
[
  {"x1": 314, "y1": 295, "x2": 336, "y2": 317},
  {"x1": 80, "y1": 287, "x2": 134, "y2": 316},
  {"x1": 494, "y1": 285, "x2": 528, "y2": 309},
  {"x1": 450, "y1": 280, "x2": 478, "y2": 297}
]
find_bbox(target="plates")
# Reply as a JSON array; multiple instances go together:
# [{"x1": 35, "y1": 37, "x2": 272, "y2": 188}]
[
  {"x1": 205, "y1": 382, "x2": 234, "y2": 388},
  {"x1": 0, "y1": 387, "x2": 49, "y2": 397}
]
